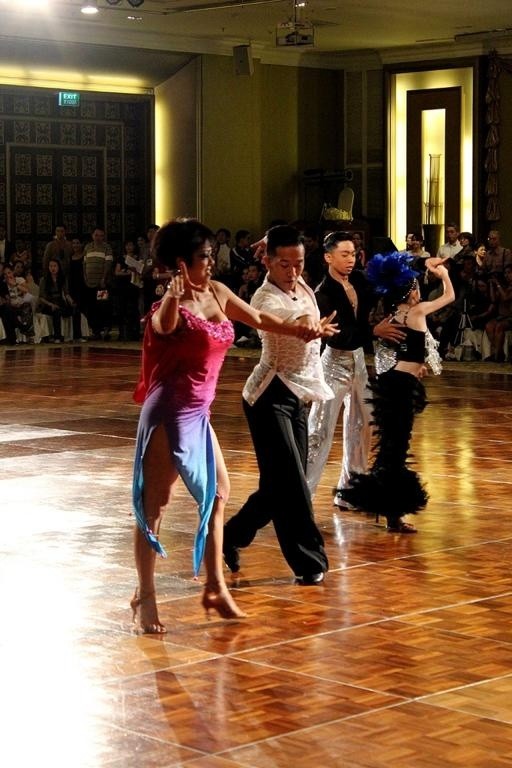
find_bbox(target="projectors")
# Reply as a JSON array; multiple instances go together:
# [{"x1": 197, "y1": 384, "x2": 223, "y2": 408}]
[{"x1": 278, "y1": 30, "x2": 313, "y2": 45}]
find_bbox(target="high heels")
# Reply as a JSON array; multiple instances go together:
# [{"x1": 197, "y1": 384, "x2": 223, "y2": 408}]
[
  {"x1": 130, "y1": 588, "x2": 167, "y2": 633},
  {"x1": 375, "y1": 512, "x2": 417, "y2": 532},
  {"x1": 202, "y1": 582, "x2": 248, "y2": 620}
]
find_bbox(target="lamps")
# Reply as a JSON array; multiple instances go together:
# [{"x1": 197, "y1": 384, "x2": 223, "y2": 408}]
[{"x1": 81, "y1": 0, "x2": 100, "y2": 15}]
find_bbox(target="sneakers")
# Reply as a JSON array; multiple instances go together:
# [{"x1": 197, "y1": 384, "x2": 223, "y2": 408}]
[{"x1": 53, "y1": 338, "x2": 89, "y2": 344}]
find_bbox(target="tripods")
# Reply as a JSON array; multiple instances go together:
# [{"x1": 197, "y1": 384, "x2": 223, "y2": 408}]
[{"x1": 452, "y1": 299, "x2": 473, "y2": 345}]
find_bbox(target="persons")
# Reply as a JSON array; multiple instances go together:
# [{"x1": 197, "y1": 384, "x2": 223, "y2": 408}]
[
  {"x1": 1, "y1": 220, "x2": 512, "y2": 363},
  {"x1": 131, "y1": 220, "x2": 455, "y2": 632}
]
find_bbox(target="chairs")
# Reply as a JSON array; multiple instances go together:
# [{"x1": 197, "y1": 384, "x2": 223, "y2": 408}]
[{"x1": 0, "y1": 277, "x2": 90, "y2": 345}]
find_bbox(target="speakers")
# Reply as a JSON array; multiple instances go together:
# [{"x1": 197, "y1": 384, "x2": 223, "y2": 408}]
[{"x1": 233, "y1": 45, "x2": 254, "y2": 80}]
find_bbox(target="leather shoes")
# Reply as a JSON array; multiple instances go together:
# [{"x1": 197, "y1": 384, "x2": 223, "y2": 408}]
[
  {"x1": 223, "y1": 525, "x2": 240, "y2": 572},
  {"x1": 302, "y1": 571, "x2": 324, "y2": 586}
]
